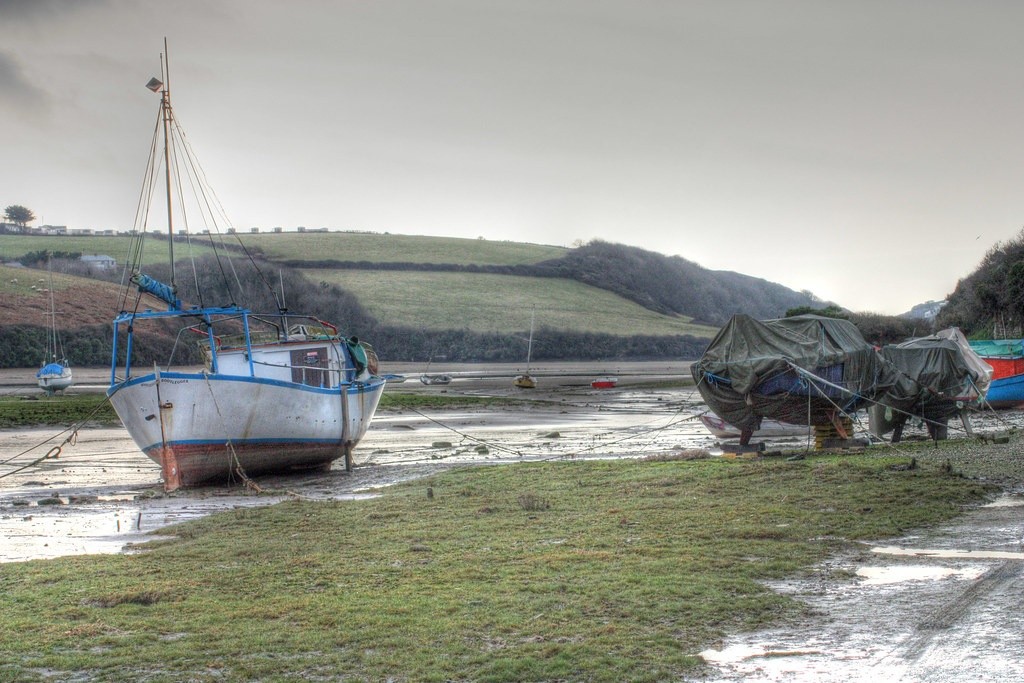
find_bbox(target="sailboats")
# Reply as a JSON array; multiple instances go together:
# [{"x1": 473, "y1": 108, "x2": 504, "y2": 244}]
[
  {"x1": 37, "y1": 248, "x2": 74, "y2": 391},
  {"x1": 515, "y1": 304, "x2": 538, "y2": 388},
  {"x1": 106, "y1": 35, "x2": 385, "y2": 494}
]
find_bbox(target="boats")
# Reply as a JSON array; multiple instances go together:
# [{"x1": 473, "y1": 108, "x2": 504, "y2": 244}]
[
  {"x1": 875, "y1": 328, "x2": 994, "y2": 418},
  {"x1": 973, "y1": 374, "x2": 1023, "y2": 409},
  {"x1": 384, "y1": 374, "x2": 407, "y2": 382},
  {"x1": 418, "y1": 374, "x2": 453, "y2": 384},
  {"x1": 592, "y1": 378, "x2": 618, "y2": 388},
  {"x1": 693, "y1": 310, "x2": 899, "y2": 424}
]
